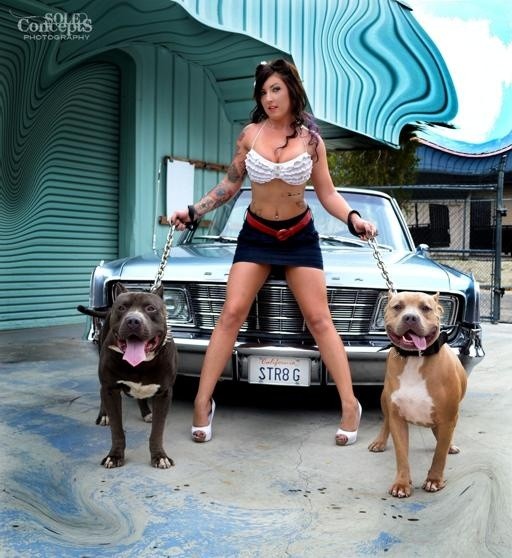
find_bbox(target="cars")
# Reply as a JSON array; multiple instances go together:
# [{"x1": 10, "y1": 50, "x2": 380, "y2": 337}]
[{"x1": 89, "y1": 186, "x2": 481, "y2": 405}]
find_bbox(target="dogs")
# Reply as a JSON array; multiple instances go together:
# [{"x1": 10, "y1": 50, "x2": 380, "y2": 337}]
[
  {"x1": 75, "y1": 281, "x2": 180, "y2": 471},
  {"x1": 366, "y1": 286, "x2": 469, "y2": 500}
]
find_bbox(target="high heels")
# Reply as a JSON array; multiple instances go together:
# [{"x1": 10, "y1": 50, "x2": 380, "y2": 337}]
[
  {"x1": 334, "y1": 400, "x2": 362, "y2": 446},
  {"x1": 191, "y1": 395, "x2": 215, "y2": 443}
]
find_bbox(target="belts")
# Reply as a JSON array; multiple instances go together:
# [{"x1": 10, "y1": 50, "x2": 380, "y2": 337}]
[{"x1": 243, "y1": 209, "x2": 316, "y2": 240}]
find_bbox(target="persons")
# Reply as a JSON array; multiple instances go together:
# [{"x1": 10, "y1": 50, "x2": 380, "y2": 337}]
[{"x1": 171, "y1": 59, "x2": 377, "y2": 446}]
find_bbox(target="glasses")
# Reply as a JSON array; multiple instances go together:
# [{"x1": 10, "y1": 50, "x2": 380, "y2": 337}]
[{"x1": 250, "y1": 58, "x2": 290, "y2": 78}]
